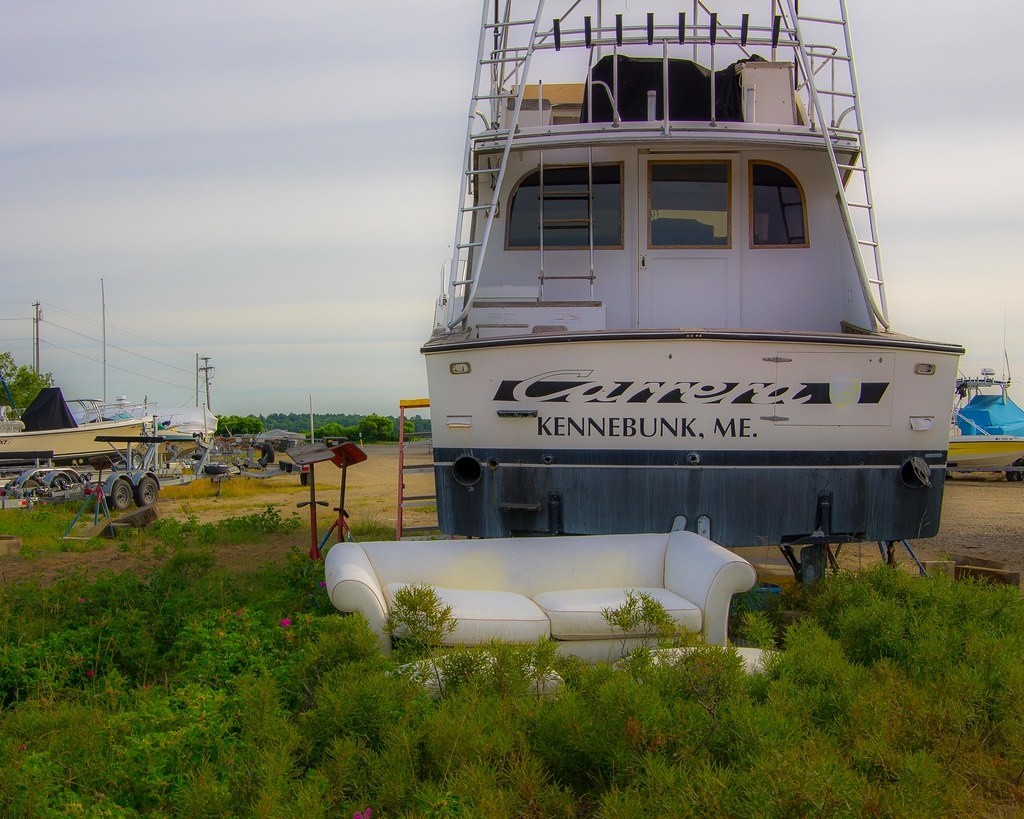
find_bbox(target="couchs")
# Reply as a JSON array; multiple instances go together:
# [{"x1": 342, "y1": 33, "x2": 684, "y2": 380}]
[{"x1": 325, "y1": 532, "x2": 756, "y2": 673}]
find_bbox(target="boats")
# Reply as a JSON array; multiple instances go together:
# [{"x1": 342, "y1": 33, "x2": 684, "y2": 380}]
[
  {"x1": 948, "y1": 368, "x2": 1024, "y2": 467},
  {"x1": 420, "y1": 1, "x2": 965, "y2": 549},
  {"x1": 0, "y1": 373, "x2": 219, "y2": 464}
]
